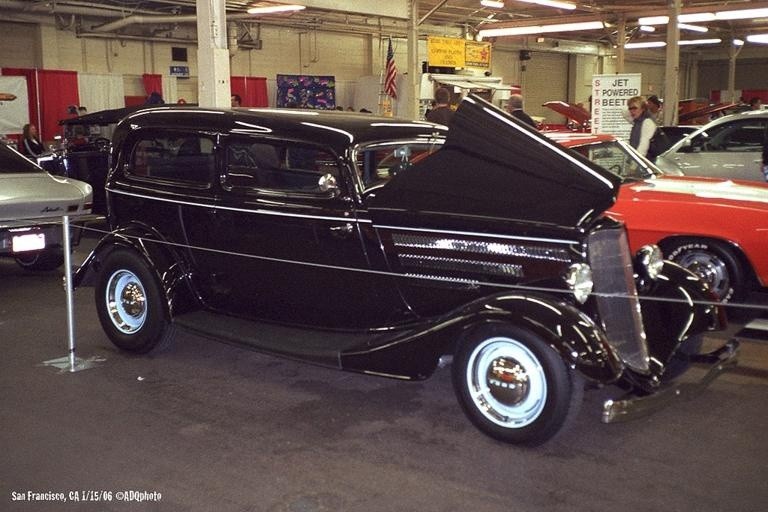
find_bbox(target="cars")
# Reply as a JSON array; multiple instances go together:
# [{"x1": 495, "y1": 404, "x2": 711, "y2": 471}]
[{"x1": 0, "y1": 136, "x2": 95, "y2": 274}]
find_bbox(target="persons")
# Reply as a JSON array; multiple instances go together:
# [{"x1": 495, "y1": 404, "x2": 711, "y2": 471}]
[
  {"x1": 646, "y1": 95, "x2": 663, "y2": 126},
  {"x1": 625, "y1": 96, "x2": 660, "y2": 177},
  {"x1": 747, "y1": 98, "x2": 761, "y2": 110},
  {"x1": 507, "y1": 93, "x2": 536, "y2": 128},
  {"x1": 427, "y1": 88, "x2": 455, "y2": 126},
  {"x1": 231, "y1": 94, "x2": 241, "y2": 107},
  {"x1": 336, "y1": 106, "x2": 370, "y2": 113},
  {"x1": 22, "y1": 124, "x2": 45, "y2": 158}
]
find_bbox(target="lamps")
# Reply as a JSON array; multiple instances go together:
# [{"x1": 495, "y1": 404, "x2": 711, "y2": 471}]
[
  {"x1": 245, "y1": 4, "x2": 306, "y2": 15},
  {"x1": 476, "y1": 0, "x2": 768, "y2": 49}
]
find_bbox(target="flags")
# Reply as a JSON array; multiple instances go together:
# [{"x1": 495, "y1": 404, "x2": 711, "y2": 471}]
[{"x1": 384, "y1": 37, "x2": 399, "y2": 101}]
